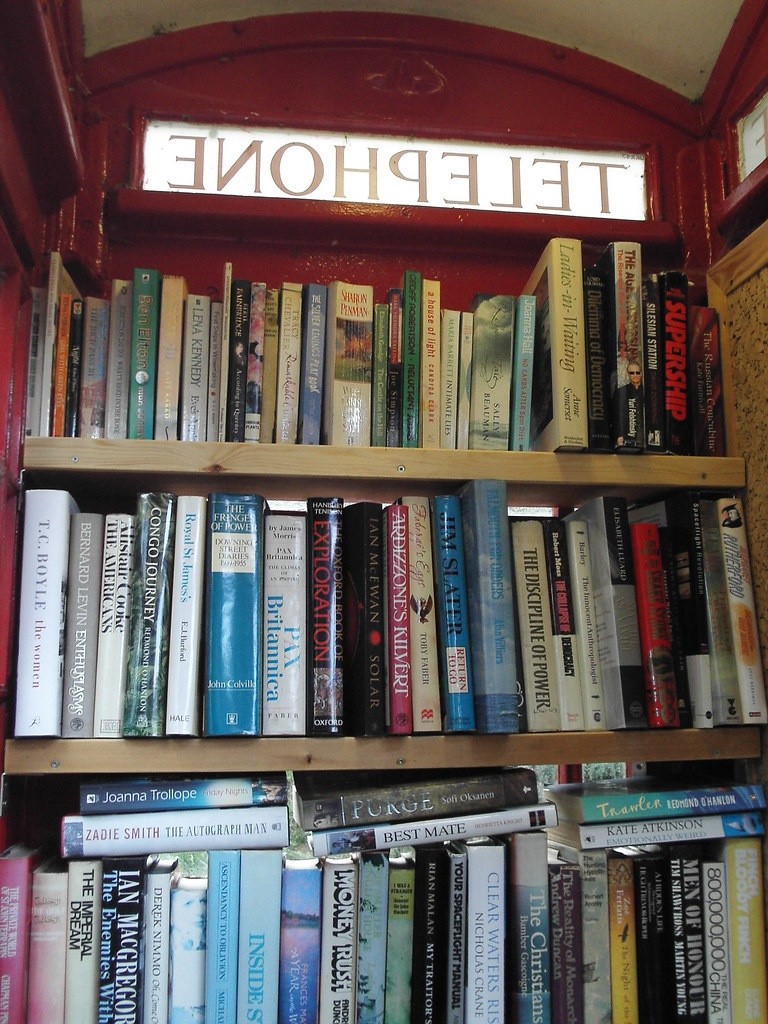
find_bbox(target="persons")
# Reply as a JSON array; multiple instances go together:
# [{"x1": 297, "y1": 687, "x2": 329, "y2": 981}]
[
  {"x1": 609, "y1": 362, "x2": 645, "y2": 448},
  {"x1": 721, "y1": 502, "x2": 742, "y2": 529},
  {"x1": 168, "y1": 886, "x2": 208, "y2": 1013}
]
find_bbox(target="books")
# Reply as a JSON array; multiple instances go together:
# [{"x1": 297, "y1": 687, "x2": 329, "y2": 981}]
[
  {"x1": 28, "y1": 224, "x2": 734, "y2": 458},
  {"x1": 10, "y1": 466, "x2": 768, "y2": 738},
  {"x1": 1, "y1": 760, "x2": 768, "y2": 1023}
]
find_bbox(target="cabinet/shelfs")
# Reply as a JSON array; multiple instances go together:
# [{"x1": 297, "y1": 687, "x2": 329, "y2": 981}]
[{"x1": 0, "y1": 8, "x2": 767, "y2": 1024}]
